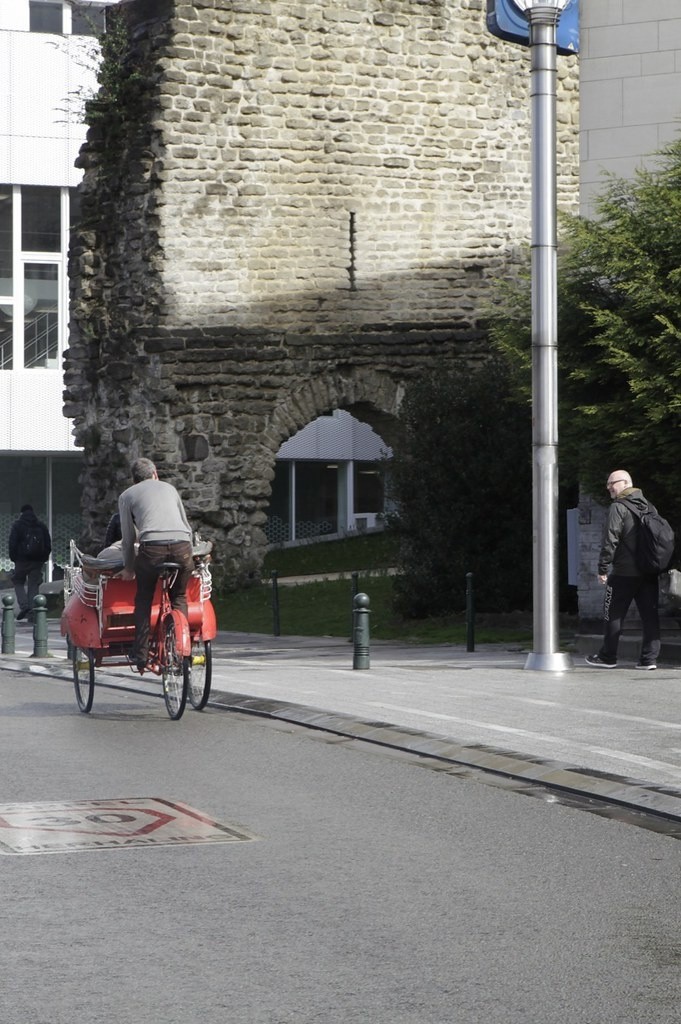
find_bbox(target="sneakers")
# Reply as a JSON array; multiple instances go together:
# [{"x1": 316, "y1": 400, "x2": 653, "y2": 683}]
[
  {"x1": 585, "y1": 653, "x2": 618, "y2": 668},
  {"x1": 635, "y1": 659, "x2": 657, "y2": 670}
]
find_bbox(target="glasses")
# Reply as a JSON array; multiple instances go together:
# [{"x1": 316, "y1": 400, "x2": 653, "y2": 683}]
[{"x1": 606, "y1": 479, "x2": 625, "y2": 487}]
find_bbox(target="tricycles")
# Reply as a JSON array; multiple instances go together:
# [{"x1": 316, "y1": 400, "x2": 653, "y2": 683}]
[{"x1": 62, "y1": 536, "x2": 218, "y2": 721}]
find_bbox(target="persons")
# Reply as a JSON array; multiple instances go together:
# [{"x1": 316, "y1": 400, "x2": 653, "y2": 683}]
[
  {"x1": 584, "y1": 469, "x2": 661, "y2": 670},
  {"x1": 97, "y1": 524, "x2": 139, "y2": 562},
  {"x1": 122, "y1": 458, "x2": 194, "y2": 675},
  {"x1": 9, "y1": 505, "x2": 52, "y2": 625}
]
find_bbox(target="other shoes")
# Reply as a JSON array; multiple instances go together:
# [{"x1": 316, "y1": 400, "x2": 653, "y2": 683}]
[
  {"x1": 128, "y1": 650, "x2": 147, "y2": 668},
  {"x1": 17, "y1": 608, "x2": 30, "y2": 620}
]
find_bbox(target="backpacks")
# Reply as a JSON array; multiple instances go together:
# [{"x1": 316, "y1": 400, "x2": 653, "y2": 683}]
[
  {"x1": 617, "y1": 499, "x2": 674, "y2": 575},
  {"x1": 21, "y1": 519, "x2": 45, "y2": 557}
]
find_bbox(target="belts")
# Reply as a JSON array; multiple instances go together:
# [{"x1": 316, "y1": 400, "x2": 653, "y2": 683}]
[{"x1": 145, "y1": 539, "x2": 184, "y2": 546}]
